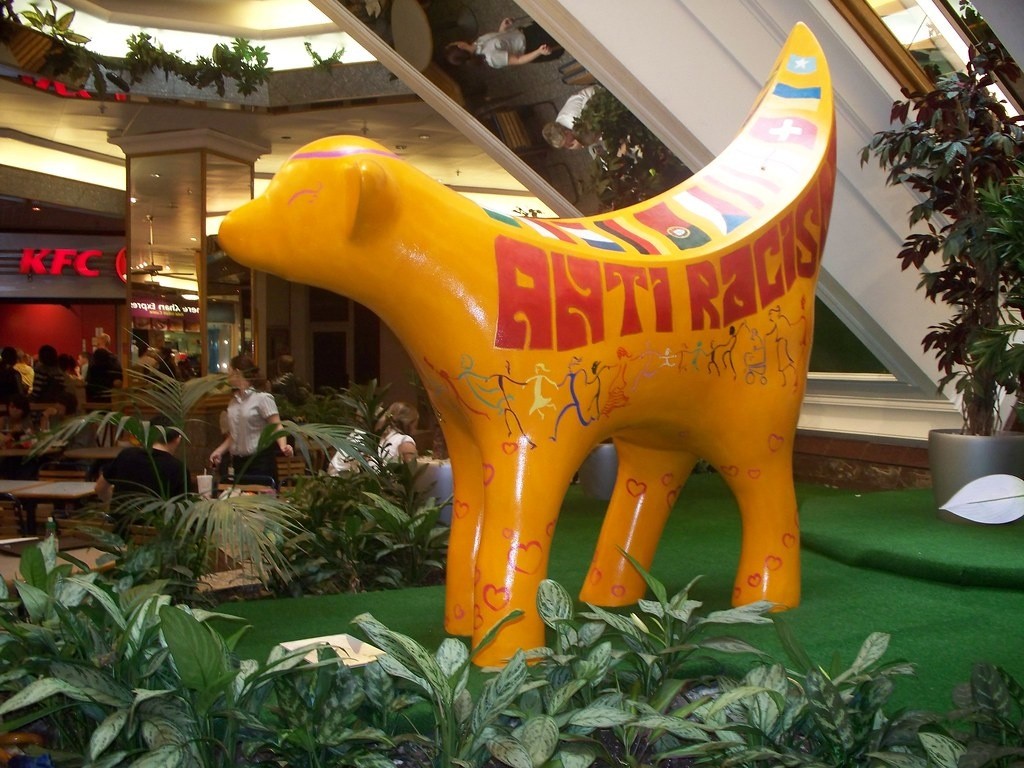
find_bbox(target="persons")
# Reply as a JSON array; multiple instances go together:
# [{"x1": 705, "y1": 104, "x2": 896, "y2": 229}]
[
  {"x1": 94, "y1": 415, "x2": 192, "y2": 530},
  {"x1": 0, "y1": 345, "x2": 79, "y2": 436},
  {"x1": 75, "y1": 353, "x2": 88, "y2": 381},
  {"x1": 86, "y1": 333, "x2": 122, "y2": 403},
  {"x1": 542, "y1": 84, "x2": 603, "y2": 150},
  {"x1": 444, "y1": 16, "x2": 563, "y2": 70},
  {"x1": 327, "y1": 404, "x2": 419, "y2": 478},
  {"x1": 209, "y1": 355, "x2": 293, "y2": 494},
  {"x1": 139, "y1": 347, "x2": 202, "y2": 382}
]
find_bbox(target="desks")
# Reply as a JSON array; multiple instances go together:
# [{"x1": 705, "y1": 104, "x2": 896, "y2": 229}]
[
  {"x1": 0, "y1": 445, "x2": 63, "y2": 481},
  {"x1": 63, "y1": 446, "x2": 128, "y2": 502},
  {"x1": 391, "y1": 0, "x2": 478, "y2": 73},
  {"x1": 0, "y1": 481, "x2": 98, "y2": 534},
  {"x1": 0, "y1": 548, "x2": 117, "y2": 622}
]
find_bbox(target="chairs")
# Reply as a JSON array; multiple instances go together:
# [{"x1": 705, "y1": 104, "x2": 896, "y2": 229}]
[
  {"x1": 278, "y1": 456, "x2": 306, "y2": 495},
  {"x1": 0, "y1": 493, "x2": 28, "y2": 537},
  {"x1": 215, "y1": 474, "x2": 275, "y2": 498},
  {"x1": 477, "y1": 102, "x2": 579, "y2": 207}
]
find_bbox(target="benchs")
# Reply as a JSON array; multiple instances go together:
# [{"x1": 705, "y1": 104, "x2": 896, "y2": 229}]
[{"x1": 54, "y1": 517, "x2": 158, "y2": 548}]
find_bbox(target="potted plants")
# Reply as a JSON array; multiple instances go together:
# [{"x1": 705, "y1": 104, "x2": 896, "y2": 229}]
[{"x1": 856, "y1": 1, "x2": 1024, "y2": 528}]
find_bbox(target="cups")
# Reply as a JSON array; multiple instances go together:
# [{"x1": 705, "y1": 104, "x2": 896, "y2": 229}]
[{"x1": 197, "y1": 475, "x2": 213, "y2": 498}]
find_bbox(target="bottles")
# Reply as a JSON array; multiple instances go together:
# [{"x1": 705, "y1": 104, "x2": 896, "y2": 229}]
[{"x1": 44, "y1": 516, "x2": 59, "y2": 554}]
